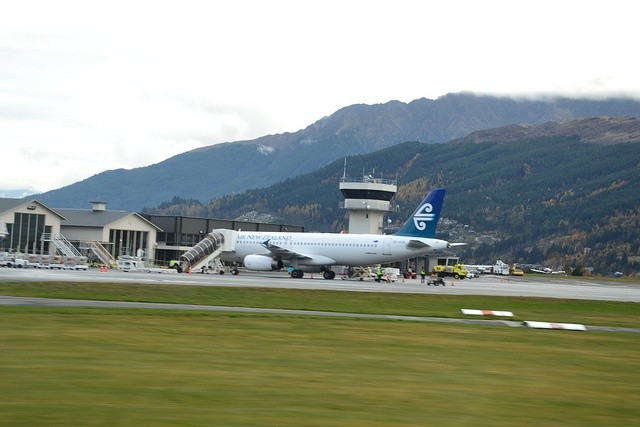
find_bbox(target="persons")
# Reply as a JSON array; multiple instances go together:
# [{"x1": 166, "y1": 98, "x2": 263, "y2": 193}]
[
  {"x1": 377, "y1": 267, "x2": 382, "y2": 283},
  {"x1": 420, "y1": 271, "x2": 426, "y2": 283}
]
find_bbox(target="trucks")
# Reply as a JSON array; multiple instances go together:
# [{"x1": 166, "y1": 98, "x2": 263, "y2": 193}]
[
  {"x1": 494, "y1": 259, "x2": 509, "y2": 275},
  {"x1": 512, "y1": 262, "x2": 524, "y2": 275},
  {"x1": 434, "y1": 263, "x2": 467, "y2": 279}
]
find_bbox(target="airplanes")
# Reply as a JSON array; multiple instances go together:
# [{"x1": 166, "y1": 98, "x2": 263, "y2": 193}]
[{"x1": 181, "y1": 187, "x2": 451, "y2": 278}]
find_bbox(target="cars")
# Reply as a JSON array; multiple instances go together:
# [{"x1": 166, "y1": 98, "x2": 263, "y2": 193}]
[{"x1": 467, "y1": 270, "x2": 479, "y2": 278}]
